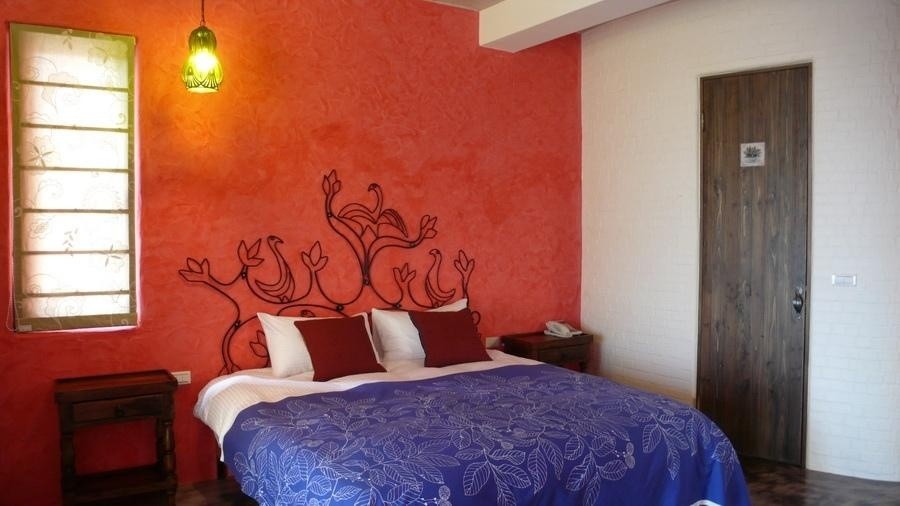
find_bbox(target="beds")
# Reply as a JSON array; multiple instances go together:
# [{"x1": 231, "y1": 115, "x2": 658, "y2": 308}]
[{"x1": 191, "y1": 347, "x2": 752, "y2": 505}]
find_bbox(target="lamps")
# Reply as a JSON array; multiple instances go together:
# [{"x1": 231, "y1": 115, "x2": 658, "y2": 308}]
[{"x1": 180, "y1": 0, "x2": 225, "y2": 94}]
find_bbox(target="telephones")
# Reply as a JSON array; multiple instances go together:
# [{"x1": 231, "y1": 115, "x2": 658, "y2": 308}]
[{"x1": 544, "y1": 321, "x2": 583, "y2": 336}]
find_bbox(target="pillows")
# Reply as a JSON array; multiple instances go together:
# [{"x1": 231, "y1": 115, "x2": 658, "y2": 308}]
[{"x1": 255, "y1": 297, "x2": 494, "y2": 383}]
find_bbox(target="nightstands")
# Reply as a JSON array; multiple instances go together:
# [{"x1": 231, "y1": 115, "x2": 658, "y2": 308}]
[
  {"x1": 500, "y1": 331, "x2": 593, "y2": 372},
  {"x1": 53, "y1": 368, "x2": 178, "y2": 506}
]
